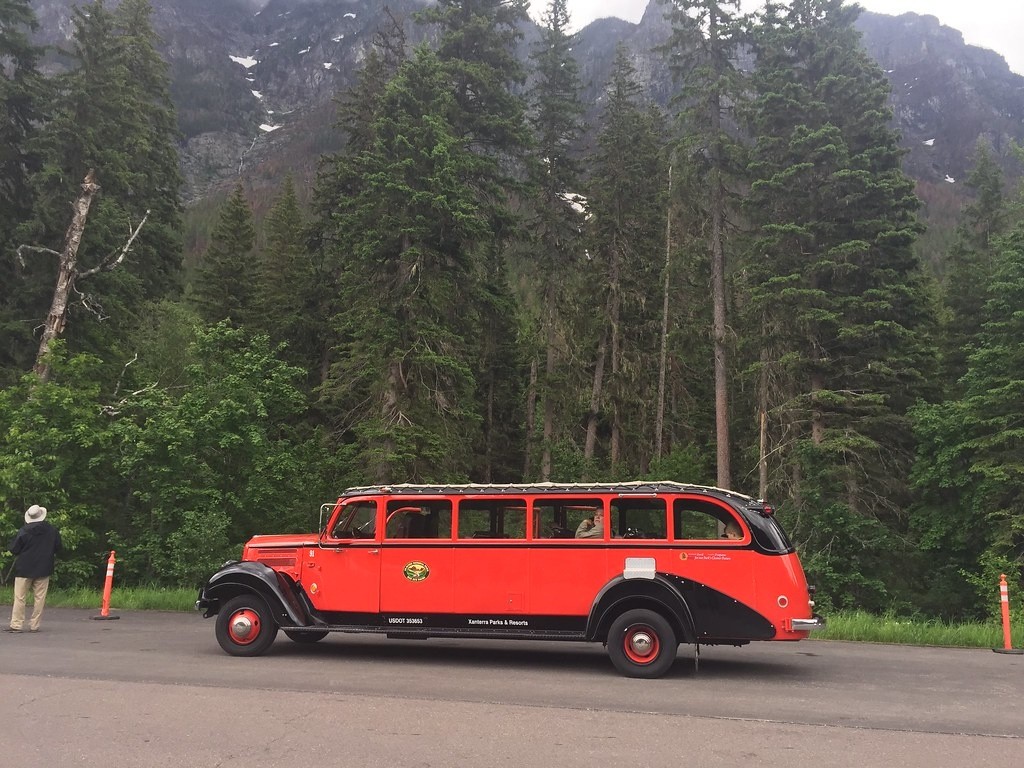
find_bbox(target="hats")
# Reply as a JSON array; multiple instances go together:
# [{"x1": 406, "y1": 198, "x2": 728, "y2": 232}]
[{"x1": 25, "y1": 505, "x2": 47, "y2": 524}]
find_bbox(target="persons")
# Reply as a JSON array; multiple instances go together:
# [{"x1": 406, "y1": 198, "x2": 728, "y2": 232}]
[
  {"x1": 575, "y1": 506, "x2": 613, "y2": 539},
  {"x1": 10, "y1": 505, "x2": 65, "y2": 633},
  {"x1": 724, "y1": 519, "x2": 743, "y2": 540}
]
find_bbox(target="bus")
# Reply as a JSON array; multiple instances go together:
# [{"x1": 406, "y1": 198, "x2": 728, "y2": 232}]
[{"x1": 198, "y1": 481, "x2": 827, "y2": 678}]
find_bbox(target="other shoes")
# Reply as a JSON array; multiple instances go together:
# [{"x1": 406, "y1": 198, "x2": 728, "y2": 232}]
[
  {"x1": 10, "y1": 627, "x2": 23, "y2": 633},
  {"x1": 28, "y1": 629, "x2": 38, "y2": 632}
]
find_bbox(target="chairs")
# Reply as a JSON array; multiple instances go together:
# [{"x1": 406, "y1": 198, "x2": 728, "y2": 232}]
[{"x1": 614, "y1": 528, "x2": 642, "y2": 539}]
